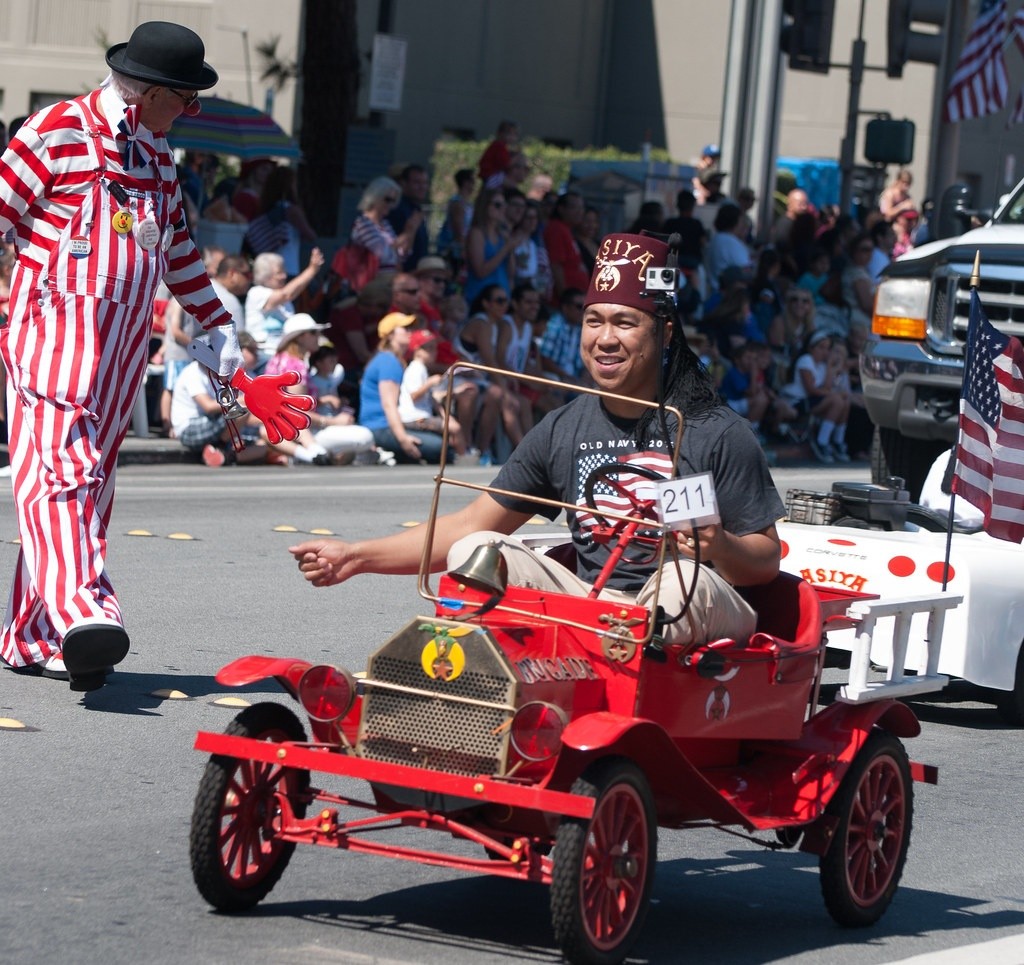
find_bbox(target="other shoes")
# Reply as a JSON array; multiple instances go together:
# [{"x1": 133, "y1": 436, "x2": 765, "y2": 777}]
[
  {"x1": 0, "y1": 654, "x2": 70, "y2": 679},
  {"x1": 62, "y1": 615, "x2": 129, "y2": 691},
  {"x1": 832, "y1": 440, "x2": 849, "y2": 463},
  {"x1": 812, "y1": 438, "x2": 836, "y2": 464},
  {"x1": 198, "y1": 439, "x2": 399, "y2": 470}
]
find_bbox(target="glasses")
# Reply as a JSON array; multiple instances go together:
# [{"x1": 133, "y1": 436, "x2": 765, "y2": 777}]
[
  {"x1": 235, "y1": 268, "x2": 253, "y2": 280},
  {"x1": 491, "y1": 202, "x2": 505, "y2": 208},
  {"x1": 143, "y1": 86, "x2": 198, "y2": 108},
  {"x1": 490, "y1": 297, "x2": 508, "y2": 304},
  {"x1": 399, "y1": 287, "x2": 418, "y2": 295},
  {"x1": 384, "y1": 197, "x2": 394, "y2": 203}
]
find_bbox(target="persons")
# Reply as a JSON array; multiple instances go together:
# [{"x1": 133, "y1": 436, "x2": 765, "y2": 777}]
[
  {"x1": 141, "y1": 149, "x2": 388, "y2": 468},
  {"x1": 0, "y1": 24, "x2": 238, "y2": 692},
  {"x1": 1, "y1": 116, "x2": 28, "y2": 472},
  {"x1": 626, "y1": 143, "x2": 920, "y2": 462},
  {"x1": 285, "y1": 231, "x2": 791, "y2": 645},
  {"x1": 313, "y1": 118, "x2": 606, "y2": 468}
]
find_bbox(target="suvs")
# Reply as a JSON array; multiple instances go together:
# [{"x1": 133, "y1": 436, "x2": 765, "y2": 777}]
[{"x1": 863, "y1": 173, "x2": 1024, "y2": 499}]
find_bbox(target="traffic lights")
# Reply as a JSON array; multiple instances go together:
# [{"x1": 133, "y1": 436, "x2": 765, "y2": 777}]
[
  {"x1": 777, "y1": 1, "x2": 837, "y2": 74},
  {"x1": 885, "y1": 0, "x2": 945, "y2": 80}
]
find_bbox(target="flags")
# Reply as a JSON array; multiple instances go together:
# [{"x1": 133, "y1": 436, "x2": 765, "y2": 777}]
[
  {"x1": 947, "y1": 0, "x2": 1010, "y2": 123},
  {"x1": 939, "y1": 286, "x2": 1024, "y2": 544}
]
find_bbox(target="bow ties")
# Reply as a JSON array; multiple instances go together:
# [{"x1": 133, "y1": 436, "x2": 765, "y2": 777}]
[{"x1": 119, "y1": 103, "x2": 158, "y2": 170}]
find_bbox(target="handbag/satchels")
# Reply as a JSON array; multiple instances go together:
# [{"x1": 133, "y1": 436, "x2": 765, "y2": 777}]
[{"x1": 333, "y1": 241, "x2": 380, "y2": 290}]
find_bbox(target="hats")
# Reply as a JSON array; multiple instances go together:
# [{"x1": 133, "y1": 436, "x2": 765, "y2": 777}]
[
  {"x1": 277, "y1": 313, "x2": 331, "y2": 353},
  {"x1": 703, "y1": 148, "x2": 723, "y2": 161},
  {"x1": 583, "y1": 232, "x2": 668, "y2": 316},
  {"x1": 105, "y1": 20, "x2": 218, "y2": 90},
  {"x1": 412, "y1": 257, "x2": 453, "y2": 279},
  {"x1": 408, "y1": 330, "x2": 441, "y2": 349},
  {"x1": 378, "y1": 312, "x2": 415, "y2": 337}
]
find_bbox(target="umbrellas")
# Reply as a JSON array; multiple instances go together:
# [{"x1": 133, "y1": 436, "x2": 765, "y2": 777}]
[{"x1": 163, "y1": 92, "x2": 306, "y2": 160}]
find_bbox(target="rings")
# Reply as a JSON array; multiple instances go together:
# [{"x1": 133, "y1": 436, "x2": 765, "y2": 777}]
[{"x1": 686, "y1": 537, "x2": 695, "y2": 548}]
